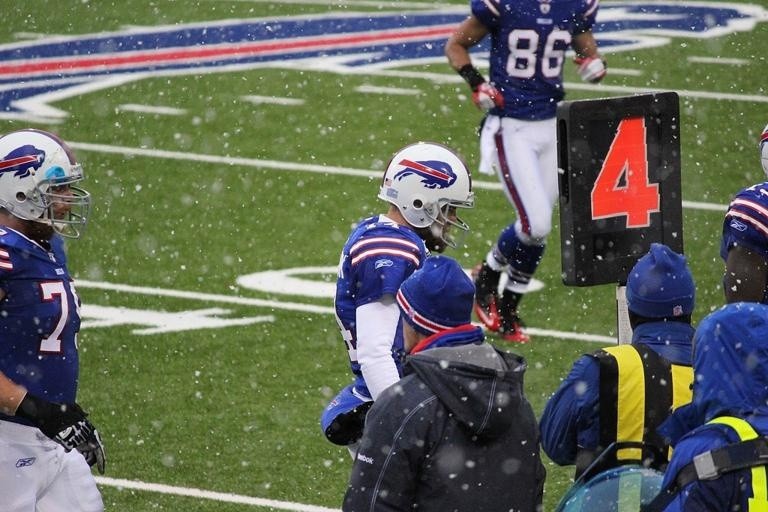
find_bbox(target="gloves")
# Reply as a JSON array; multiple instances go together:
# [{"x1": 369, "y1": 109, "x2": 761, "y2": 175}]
[
  {"x1": 13, "y1": 391, "x2": 105, "y2": 473},
  {"x1": 574, "y1": 53, "x2": 606, "y2": 83},
  {"x1": 471, "y1": 81, "x2": 506, "y2": 112}
]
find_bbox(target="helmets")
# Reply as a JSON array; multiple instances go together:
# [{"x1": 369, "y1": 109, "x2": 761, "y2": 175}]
[
  {"x1": 378, "y1": 141, "x2": 474, "y2": 253},
  {"x1": 0, "y1": 129, "x2": 90, "y2": 238}
]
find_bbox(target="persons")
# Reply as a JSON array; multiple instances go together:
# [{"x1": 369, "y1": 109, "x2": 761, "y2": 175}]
[
  {"x1": 443, "y1": 0, "x2": 604, "y2": 342},
  {"x1": 342, "y1": 256, "x2": 547, "y2": 510},
  {"x1": 0, "y1": 419, "x2": 107, "y2": 511},
  {"x1": 536, "y1": 247, "x2": 696, "y2": 476},
  {"x1": 320, "y1": 139, "x2": 474, "y2": 461},
  {"x1": 663, "y1": 301, "x2": 765, "y2": 510},
  {"x1": 724, "y1": 124, "x2": 766, "y2": 300},
  {"x1": 0, "y1": 129, "x2": 106, "y2": 477}
]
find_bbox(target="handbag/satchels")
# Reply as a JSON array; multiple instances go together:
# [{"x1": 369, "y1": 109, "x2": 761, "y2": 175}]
[{"x1": 319, "y1": 384, "x2": 374, "y2": 446}]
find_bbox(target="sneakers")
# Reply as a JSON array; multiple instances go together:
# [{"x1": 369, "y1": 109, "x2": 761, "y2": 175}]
[{"x1": 471, "y1": 264, "x2": 530, "y2": 343}]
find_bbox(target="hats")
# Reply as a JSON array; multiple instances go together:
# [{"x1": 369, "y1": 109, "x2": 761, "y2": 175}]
[
  {"x1": 625, "y1": 243, "x2": 695, "y2": 319},
  {"x1": 396, "y1": 256, "x2": 475, "y2": 334}
]
